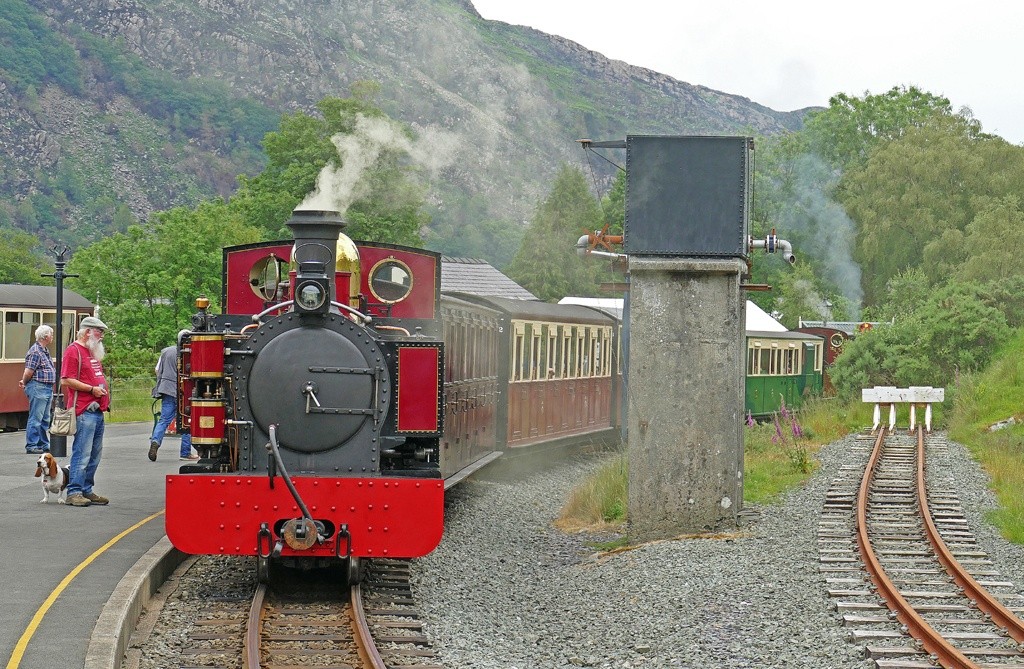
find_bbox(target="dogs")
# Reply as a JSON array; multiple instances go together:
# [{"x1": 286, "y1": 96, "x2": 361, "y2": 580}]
[{"x1": 35, "y1": 452, "x2": 71, "y2": 505}]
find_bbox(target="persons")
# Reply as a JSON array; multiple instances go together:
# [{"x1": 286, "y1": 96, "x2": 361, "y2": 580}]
[
  {"x1": 19, "y1": 325, "x2": 56, "y2": 454},
  {"x1": 60, "y1": 317, "x2": 109, "y2": 507},
  {"x1": 148, "y1": 330, "x2": 200, "y2": 461}
]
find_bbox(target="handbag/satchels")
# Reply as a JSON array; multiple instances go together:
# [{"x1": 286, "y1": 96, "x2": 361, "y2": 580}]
[{"x1": 49, "y1": 406, "x2": 77, "y2": 437}]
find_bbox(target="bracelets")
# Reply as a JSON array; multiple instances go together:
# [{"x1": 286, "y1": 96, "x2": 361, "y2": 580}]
[{"x1": 89, "y1": 386, "x2": 94, "y2": 394}]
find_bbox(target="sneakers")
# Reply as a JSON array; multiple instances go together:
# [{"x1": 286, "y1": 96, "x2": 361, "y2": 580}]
[
  {"x1": 65, "y1": 492, "x2": 91, "y2": 507},
  {"x1": 83, "y1": 493, "x2": 109, "y2": 505}
]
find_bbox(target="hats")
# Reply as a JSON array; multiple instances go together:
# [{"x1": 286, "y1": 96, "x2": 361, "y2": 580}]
[{"x1": 80, "y1": 317, "x2": 109, "y2": 330}]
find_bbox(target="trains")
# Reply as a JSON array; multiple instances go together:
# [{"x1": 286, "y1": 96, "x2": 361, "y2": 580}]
[{"x1": 164, "y1": 208, "x2": 892, "y2": 587}]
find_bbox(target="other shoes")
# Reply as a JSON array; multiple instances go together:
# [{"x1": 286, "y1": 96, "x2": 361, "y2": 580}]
[
  {"x1": 44, "y1": 447, "x2": 50, "y2": 453},
  {"x1": 148, "y1": 440, "x2": 159, "y2": 462},
  {"x1": 26, "y1": 448, "x2": 43, "y2": 454},
  {"x1": 179, "y1": 454, "x2": 200, "y2": 462}
]
